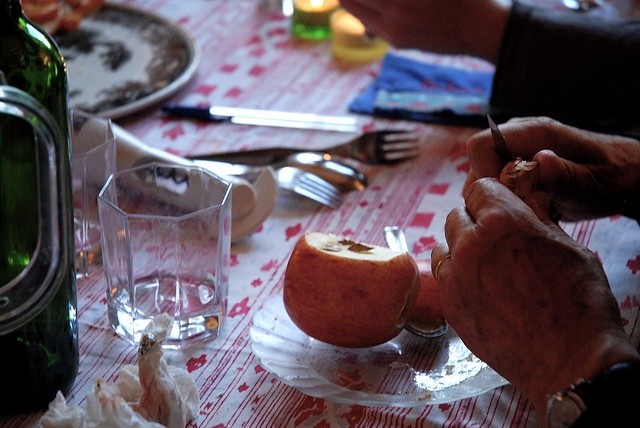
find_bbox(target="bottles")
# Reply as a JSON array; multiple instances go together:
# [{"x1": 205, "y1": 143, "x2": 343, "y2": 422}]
[{"x1": 0, "y1": 0, "x2": 80, "y2": 414}]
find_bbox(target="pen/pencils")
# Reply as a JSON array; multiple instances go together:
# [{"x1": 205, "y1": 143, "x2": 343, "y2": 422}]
[{"x1": 159, "y1": 102, "x2": 357, "y2": 133}]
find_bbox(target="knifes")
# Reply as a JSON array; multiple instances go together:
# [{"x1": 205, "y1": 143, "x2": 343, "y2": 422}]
[{"x1": 485, "y1": 112, "x2": 517, "y2": 176}]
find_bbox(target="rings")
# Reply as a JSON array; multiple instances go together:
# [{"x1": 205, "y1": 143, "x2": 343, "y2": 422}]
[{"x1": 435, "y1": 254, "x2": 451, "y2": 278}]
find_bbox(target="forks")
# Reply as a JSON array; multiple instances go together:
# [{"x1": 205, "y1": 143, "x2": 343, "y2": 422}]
[
  {"x1": 206, "y1": 166, "x2": 343, "y2": 211},
  {"x1": 186, "y1": 127, "x2": 422, "y2": 166}
]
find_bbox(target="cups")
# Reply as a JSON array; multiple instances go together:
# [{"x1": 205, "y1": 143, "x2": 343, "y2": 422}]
[
  {"x1": 97, "y1": 164, "x2": 232, "y2": 344},
  {"x1": 70, "y1": 110, "x2": 117, "y2": 279}
]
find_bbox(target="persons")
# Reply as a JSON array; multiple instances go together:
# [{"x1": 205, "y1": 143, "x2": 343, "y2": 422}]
[
  {"x1": 431, "y1": 116, "x2": 639, "y2": 428},
  {"x1": 339, "y1": 0, "x2": 640, "y2": 137}
]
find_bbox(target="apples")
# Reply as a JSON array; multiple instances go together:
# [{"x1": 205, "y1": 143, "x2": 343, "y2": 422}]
[
  {"x1": 284, "y1": 232, "x2": 420, "y2": 348},
  {"x1": 413, "y1": 259, "x2": 443, "y2": 321}
]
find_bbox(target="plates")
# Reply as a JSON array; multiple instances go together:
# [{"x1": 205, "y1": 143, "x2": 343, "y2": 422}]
[
  {"x1": 56, "y1": 4, "x2": 201, "y2": 121},
  {"x1": 248, "y1": 295, "x2": 511, "y2": 408}
]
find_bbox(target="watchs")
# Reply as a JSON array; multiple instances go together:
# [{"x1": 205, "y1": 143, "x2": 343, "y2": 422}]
[{"x1": 544, "y1": 380, "x2": 591, "y2": 428}]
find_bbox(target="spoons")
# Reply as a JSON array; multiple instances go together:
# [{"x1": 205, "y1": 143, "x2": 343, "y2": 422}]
[
  {"x1": 383, "y1": 226, "x2": 449, "y2": 338},
  {"x1": 278, "y1": 152, "x2": 369, "y2": 191}
]
